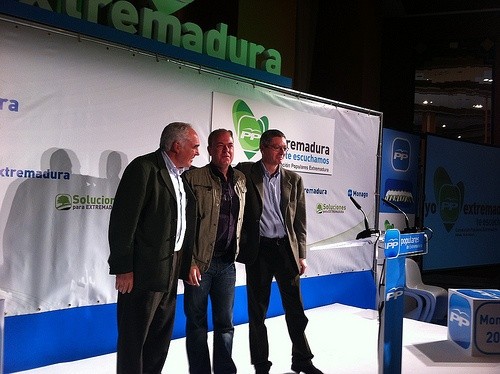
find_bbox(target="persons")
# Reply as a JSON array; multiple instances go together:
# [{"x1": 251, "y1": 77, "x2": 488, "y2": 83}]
[
  {"x1": 107, "y1": 122, "x2": 200, "y2": 374},
  {"x1": 232, "y1": 129, "x2": 323, "y2": 374},
  {"x1": 183, "y1": 129, "x2": 247, "y2": 374}
]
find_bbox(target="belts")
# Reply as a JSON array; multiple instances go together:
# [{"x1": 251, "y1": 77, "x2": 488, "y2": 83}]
[{"x1": 259, "y1": 236, "x2": 290, "y2": 246}]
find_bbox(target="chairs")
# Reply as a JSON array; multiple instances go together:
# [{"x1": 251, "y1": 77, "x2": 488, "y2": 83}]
[{"x1": 404, "y1": 258, "x2": 448, "y2": 325}]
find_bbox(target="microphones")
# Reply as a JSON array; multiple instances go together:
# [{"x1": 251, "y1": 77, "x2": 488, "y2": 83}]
[
  {"x1": 381, "y1": 198, "x2": 417, "y2": 235},
  {"x1": 349, "y1": 197, "x2": 379, "y2": 240}
]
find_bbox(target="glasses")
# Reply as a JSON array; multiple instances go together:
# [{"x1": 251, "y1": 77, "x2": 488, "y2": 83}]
[{"x1": 265, "y1": 144, "x2": 288, "y2": 152}]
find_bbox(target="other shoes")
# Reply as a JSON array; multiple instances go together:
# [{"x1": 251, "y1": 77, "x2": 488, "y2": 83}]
[{"x1": 291, "y1": 362, "x2": 323, "y2": 374}]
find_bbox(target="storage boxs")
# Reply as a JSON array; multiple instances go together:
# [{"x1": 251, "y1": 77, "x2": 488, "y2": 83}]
[{"x1": 447, "y1": 288, "x2": 500, "y2": 358}]
